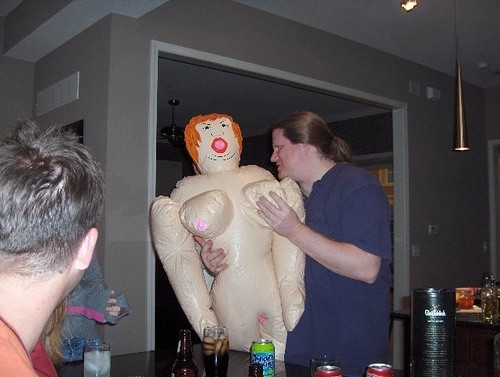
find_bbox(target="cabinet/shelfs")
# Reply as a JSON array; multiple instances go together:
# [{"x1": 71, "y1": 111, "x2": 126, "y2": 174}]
[{"x1": 390, "y1": 305, "x2": 500, "y2": 377}]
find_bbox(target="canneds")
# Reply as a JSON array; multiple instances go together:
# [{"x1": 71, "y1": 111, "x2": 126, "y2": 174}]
[
  {"x1": 366, "y1": 363, "x2": 394, "y2": 377},
  {"x1": 313, "y1": 365, "x2": 343, "y2": 377},
  {"x1": 250, "y1": 339, "x2": 275, "y2": 377}
]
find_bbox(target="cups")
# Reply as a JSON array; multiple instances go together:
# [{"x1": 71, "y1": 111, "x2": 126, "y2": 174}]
[
  {"x1": 83, "y1": 342, "x2": 111, "y2": 377},
  {"x1": 202, "y1": 326, "x2": 229, "y2": 377},
  {"x1": 454, "y1": 287, "x2": 476, "y2": 311},
  {"x1": 311, "y1": 357, "x2": 342, "y2": 377}
]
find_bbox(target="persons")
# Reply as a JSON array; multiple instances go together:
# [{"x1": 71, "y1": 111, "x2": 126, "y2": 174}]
[
  {"x1": 194, "y1": 111, "x2": 392, "y2": 377},
  {"x1": 0, "y1": 118, "x2": 130, "y2": 377}
]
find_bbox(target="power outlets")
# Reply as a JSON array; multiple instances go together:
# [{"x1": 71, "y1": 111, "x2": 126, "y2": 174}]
[{"x1": 428, "y1": 224, "x2": 437, "y2": 235}]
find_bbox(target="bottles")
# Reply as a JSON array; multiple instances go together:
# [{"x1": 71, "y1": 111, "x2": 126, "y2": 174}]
[
  {"x1": 170, "y1": 330, "x2": 198, "y2": 377},
  {"x1": 248, "y1": 363, "x2": 263, "y2": 377},
  {"x1": 480, "y1": 272, "x2": 499, "y2": 324}
]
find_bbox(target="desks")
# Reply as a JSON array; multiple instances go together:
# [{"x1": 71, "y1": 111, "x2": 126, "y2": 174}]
[{"x1": 53, "y1": 344, "x2": 312, "y2": 377}]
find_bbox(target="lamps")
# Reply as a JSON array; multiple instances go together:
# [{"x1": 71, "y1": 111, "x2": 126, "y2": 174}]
[
  {"x1": 161, "y1": 100, "x2": 185, "y2": 144},
  {"x1": 452, "y1": 1, "x2": 471, "y2": 152}
]
